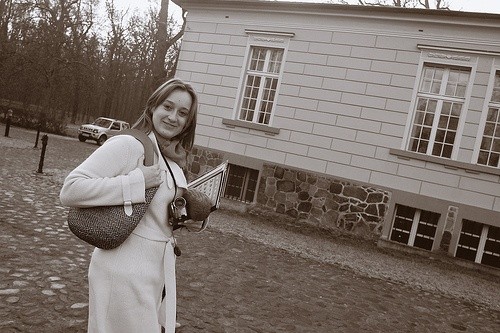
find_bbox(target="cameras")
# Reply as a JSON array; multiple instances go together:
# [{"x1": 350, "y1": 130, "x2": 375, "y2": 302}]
[{"x1": 168, "y1": 197, "x2": 189, "y2": 222}]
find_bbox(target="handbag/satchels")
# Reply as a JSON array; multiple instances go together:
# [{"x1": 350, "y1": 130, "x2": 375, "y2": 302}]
[{"x1": 68, "y1": 129, "x2": 159, "y2": 250}]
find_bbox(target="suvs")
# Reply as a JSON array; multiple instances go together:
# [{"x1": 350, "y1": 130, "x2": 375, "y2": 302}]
[{"x1": 77, "y1": 116, "x2": 131, "y2": 146}]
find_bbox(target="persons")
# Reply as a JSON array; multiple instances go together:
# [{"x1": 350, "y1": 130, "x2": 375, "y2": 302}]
[{"x1": 60, "y1": 78, "x2": 211, "y2": 333}]
